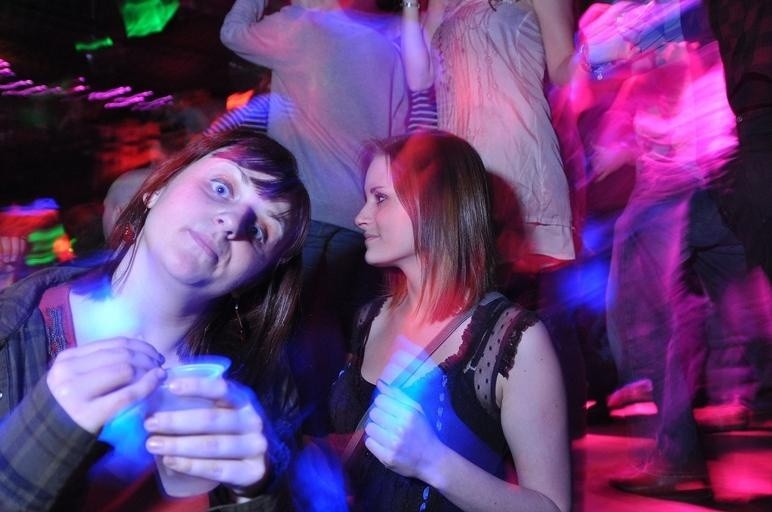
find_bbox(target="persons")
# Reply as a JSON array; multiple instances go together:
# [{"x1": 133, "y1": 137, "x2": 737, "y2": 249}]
[
  {"x1": 183, "y1": 74, "x2": 434, "y2": 148},
  {"x1": 398, "y1": 0, "x2": 582, "y2": 307},
  {"x1": 321, "y1": 132, "x2": 571, "y2": 512},
  {"x1": 0, "y1": 137, "x2": 343, "y2": 512},
  {"x1": 571, "y1": 4, "x2": 772, "y2": 501},
  {"x1": 216, "y1": 0, "x2": 412, "y2": 267},
  {"x1": 101, "y1": 170, "x2": 163, "y2": 248}
]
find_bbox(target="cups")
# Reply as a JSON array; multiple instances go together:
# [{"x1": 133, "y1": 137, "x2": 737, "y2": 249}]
[{"x1": 139, "y1": 362, "x2": 225, "y2": 498}]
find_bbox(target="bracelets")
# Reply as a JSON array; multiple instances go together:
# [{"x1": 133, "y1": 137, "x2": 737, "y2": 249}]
[{"x1": 400, "y1": 1, "x2": 420, "y2": 8}]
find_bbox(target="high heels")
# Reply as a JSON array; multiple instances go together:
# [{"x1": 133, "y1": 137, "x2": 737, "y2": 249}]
[{"x1": 606, "y1": 465, "x2": 709, "y2": 494}]
